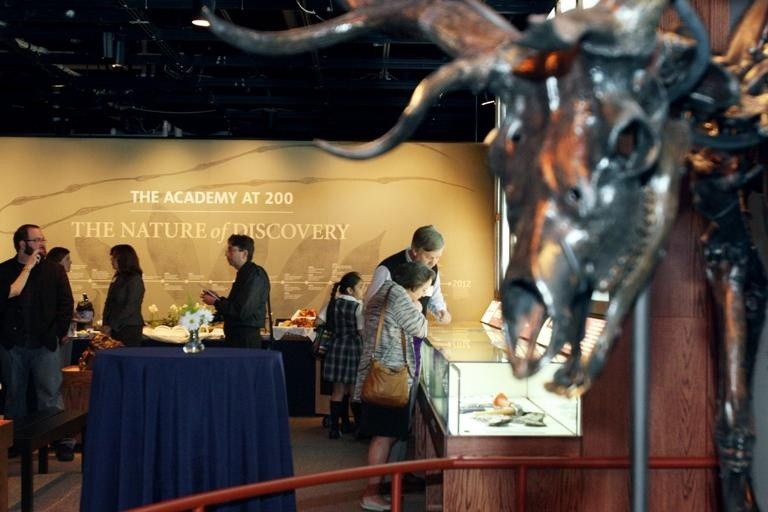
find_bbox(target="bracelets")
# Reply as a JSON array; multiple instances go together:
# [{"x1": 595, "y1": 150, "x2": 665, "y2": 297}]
[{"x1": 23, "y1": 264, "x2": 32, "y2": 273}]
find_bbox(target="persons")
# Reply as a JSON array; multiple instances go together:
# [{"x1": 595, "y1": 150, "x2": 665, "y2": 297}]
[
  {"x1": 0, "y1": 224, "x2": 76, "y2": 462},
  {"x1": 200, "y1": 234, "x2": 271, "y2": 348},
  {"x1": 99, "y1": 244, "x2": 145, "y2": 347},
  {"x1": 354, "y1": 261, "x2": 436, "y2": 512},
  {"x1": 313, "y1": 272, "x2": 365, "y2": 439},
  {"x1": 44, "y1": 246, "x2": 74, "y2": 368},
  {"x1": 361, "y1": 224, "x2": 452, "y2": 504}
]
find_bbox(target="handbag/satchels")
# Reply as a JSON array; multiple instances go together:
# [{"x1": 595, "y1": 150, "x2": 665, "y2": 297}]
[
  {"x1": 362, "y1": 361, "x2": 410, "y2": 410},
  {"x1": 312, "y1": 324, "x2": 333, "y2": 360}
]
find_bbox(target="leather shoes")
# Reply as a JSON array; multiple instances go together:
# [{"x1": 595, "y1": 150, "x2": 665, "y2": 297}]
[
  {"x1": 359, "y1": 492, "x2": 404, "y2": 511},
  {"x1": 329, "y1": 423, "x2": 354, "y2": 440}
]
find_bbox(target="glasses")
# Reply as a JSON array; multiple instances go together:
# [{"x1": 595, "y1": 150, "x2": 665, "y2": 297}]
[{"x1": 24, "y1": 238, "x2": 47, "y2": 245}]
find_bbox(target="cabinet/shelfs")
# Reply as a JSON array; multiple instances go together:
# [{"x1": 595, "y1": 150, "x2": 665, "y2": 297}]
[{"x1": 409, "y1": 203, "x2": 717, "y2": 512}]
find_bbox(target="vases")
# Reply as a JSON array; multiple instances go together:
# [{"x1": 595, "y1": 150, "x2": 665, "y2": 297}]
[{"x1": 183, "y1": 329, "x2": 205, "y2": 353}]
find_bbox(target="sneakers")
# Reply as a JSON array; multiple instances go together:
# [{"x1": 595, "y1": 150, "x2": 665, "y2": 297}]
[
  {"x1": 7, "y1": 443, "x2": 38, "y2": 459},
  {"x1": 405, "y1": 473, "x2": 426, "y2": 492},
  {"x1": 55, "y1": 443, "x2": 74, "y2": 461}
]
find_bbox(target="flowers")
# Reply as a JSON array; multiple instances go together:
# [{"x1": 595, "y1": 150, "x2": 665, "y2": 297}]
[{"x1": 178, "y1": 287, "x2": 215, "y2": 332}]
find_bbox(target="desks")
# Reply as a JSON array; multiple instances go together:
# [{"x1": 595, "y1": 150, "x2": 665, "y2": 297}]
[{"x1": 79, "y1": 346, "x2": 296, "y2": 512}]
